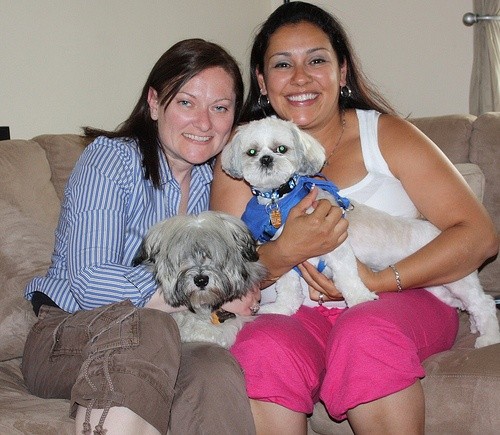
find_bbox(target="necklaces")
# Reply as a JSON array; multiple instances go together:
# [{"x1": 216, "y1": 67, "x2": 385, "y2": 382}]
[{"x1": 319, "y1": 114, "x2": 346, "y2": 170}]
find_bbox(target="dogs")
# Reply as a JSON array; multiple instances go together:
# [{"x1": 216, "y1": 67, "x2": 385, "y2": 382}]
[
  {"x1": 130, "y1": 209, "x2": 269, "y2": 349},
  {"x1": 220, "y1": 115, "x2": 500, "y2": 348}
]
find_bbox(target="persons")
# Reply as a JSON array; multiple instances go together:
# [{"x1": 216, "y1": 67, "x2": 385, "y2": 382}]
[
  {"x1": 210, "y1": 0, "x2": 500, "y2": 435},
  {"x1": 20, "y1": 37, "x2": 262, "y2": 435}
]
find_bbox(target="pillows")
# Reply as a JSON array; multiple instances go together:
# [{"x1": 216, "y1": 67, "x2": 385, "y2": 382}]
[{"x1": 421, "y1": 161, "x2": 485, "y2": 221}]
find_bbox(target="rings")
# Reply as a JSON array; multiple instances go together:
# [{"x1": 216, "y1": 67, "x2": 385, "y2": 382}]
[
  {"x1": 318, "y1": 293, "x2": 324, "y2": 305},
  {"x1": 249, "y1": 301, "x2": 261, "y2": 313}
]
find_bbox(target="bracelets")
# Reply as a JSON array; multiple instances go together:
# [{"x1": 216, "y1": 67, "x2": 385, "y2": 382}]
[{"x1": 388, "y1": 264, "x2": 403, "y2": 292}]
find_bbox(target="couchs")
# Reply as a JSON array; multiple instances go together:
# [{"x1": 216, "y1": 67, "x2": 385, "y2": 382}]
[{"x1": 0, "y1": 111, "x2": 500, "y2": 435}]
